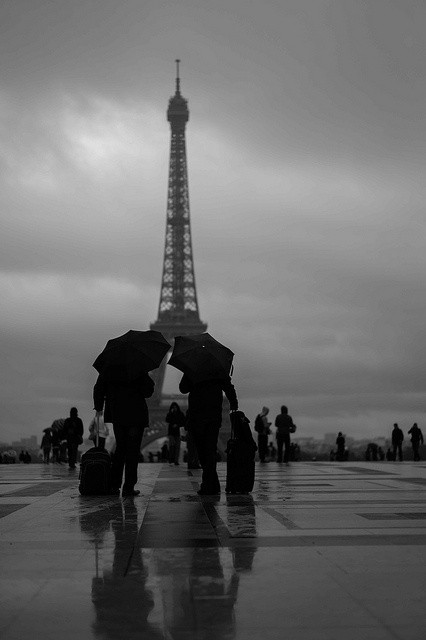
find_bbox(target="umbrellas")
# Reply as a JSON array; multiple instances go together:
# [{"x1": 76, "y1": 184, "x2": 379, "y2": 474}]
[
  {"x1": 123, "y1": 490, "x2": 140, "y2": 497},
  {"x1": 197, "y1": 490, "x2": 220, "y2": 494},
  {"x1": 111, "y1": 489, "x2": 120, "y2": 495}
]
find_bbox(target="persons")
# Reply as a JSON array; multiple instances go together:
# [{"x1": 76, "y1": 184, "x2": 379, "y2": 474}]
[
  {"x1": 408, "y1": 424, "x2": 423, "y2": 461},
  {"x1": 258, "y1": 407, "x2": 272, "y2": 462},
  {"x1": 392, "y1": 424, "x2": 403, "y2": 461},
  {"x1": 52, "y1": 427, "x2": 68, "y2": 464},
  {"x1": 19, "y1": 451, "x2": 30, "y2": 464},
  {"x1": 89, "y1": 409, "x2": 109, "y2": 448},
  {"x1": 365, "y1": 447, "x2": 394, "y2": 460},
  {"x1": 336, "y1": 432, "x2": 345, "y2": 461},
  {"x1": 166, "y1": 402, "x2": 184, "y2": 465},
  {"x1": 275, "y1": 406, "x2": 292, "y2": 462},
  {"x1": 41, "y1": 431, "x2": 52, "y2": 463},
  {"x1": 185, "y1": 409, "x2": 201, "y2": 469},
  {"x1": 179, "y1": 373, "x2": 237, "y2": 494},
  {"x1": 60, "y1": 408, "x2": 83, "y2": 467},
  {"x1": 93, "y1": 373, "x2": 155, "y2": 496}
]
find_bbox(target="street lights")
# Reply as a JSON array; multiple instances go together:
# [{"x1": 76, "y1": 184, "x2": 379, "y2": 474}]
[
  {"x1": 229, "y1": 411, "x2": 257, "y2": 454},
  {"x1": 89, "y1": 425, "x2": 94, "y2": 440}
]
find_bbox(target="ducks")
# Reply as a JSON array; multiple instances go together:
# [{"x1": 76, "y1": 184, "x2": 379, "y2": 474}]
[
  {"x1": 79, "y1": 414, "x2": 112, "y2": 496},
  {"x1": 225, "y1": 409, "x2": 255, "y2": 495}
]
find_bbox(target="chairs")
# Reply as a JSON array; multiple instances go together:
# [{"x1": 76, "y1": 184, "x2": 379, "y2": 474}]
[
  {"x1": 175, "y1": 462, "x2": 179, "y2": 465},
  {"x1": 168, "y1": 459, "x2": 173, "y2": 463}
]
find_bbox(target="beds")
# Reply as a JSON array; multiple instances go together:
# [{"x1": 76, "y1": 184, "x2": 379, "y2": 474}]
[
  {"x1": 43, "y1": 428, "x2": 52, "y2": 432},
  {"x1": 51, "y1": 420, "x2": 65, "y2": 430},
  {"x1": 167, "y1": 333, "x2": 235, "y2": 380},
  {"x1": 92, "y1": 330, "x2": 171, "y2": 378}
]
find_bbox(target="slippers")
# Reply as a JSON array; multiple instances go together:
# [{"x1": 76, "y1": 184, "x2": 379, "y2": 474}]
[{"x1": 255, "y1": 414, "x2": 264, "y2": 432}]
